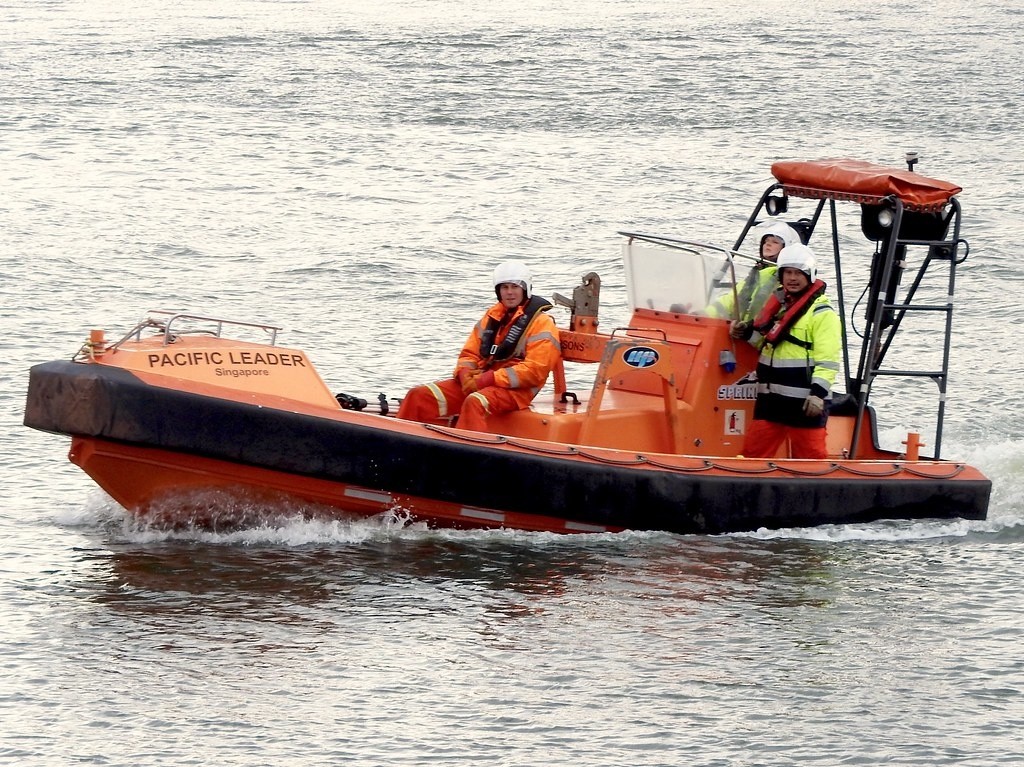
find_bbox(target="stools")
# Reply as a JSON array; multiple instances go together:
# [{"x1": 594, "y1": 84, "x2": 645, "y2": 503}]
[{"x1": 737, "y1": 380, "x2": 856, "y2": 458}]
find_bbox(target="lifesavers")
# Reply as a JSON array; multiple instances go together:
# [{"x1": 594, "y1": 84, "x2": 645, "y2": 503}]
[
  {"x1": 477, "y1": 296, "x2": 555, "y2": 360},
  {"x1": 754, "y1": 278, "x2": 827, "y2": 346}
]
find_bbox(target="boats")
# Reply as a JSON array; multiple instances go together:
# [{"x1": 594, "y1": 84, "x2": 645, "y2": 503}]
[{"x1": 18, "y1": 159, "x2": 992, "y2": 537}]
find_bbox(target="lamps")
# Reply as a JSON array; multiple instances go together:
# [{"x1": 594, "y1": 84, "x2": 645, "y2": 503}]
[
  {"x1": 858, "y1": 200, "x2": 951, "y2": 242},
  {"x1": 765, "y1": 194, "x2": 789, "y2": 216}
]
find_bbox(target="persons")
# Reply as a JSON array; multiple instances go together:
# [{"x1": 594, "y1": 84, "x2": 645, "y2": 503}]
[
  {"x1": 727, "y1": 243, "x2": 842, "y2": 460},
  {"x1": 395, "y1": 261, "x2": 561, "y2": 434},
  {"x1": 691, "y1": 221, "x2": 800, "y2": 322}
]
